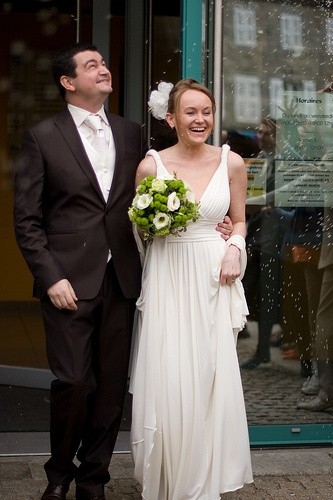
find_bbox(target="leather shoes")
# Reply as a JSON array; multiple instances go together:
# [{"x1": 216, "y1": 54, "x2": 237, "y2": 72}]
[{"x1": 41, "y1": 481, "x2": 71, "y2": 500}]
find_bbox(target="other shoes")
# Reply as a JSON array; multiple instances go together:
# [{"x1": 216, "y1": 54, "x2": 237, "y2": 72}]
[
  {"x1": 240, "y1": 350, "x2": 270, "y2": 370},
  {"x1": 296, "y1": 396, "x2": 332, "y2": 412},
  {"x1": 300, "y1": 376, "x2": 320, "y2": 395}
]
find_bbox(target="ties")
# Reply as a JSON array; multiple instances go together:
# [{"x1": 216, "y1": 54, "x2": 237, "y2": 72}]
[{"x1": 83, "y1": 115, "x2": 109, "y2": 152}]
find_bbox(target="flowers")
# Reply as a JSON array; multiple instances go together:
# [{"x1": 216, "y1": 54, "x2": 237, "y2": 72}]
[
  {"x1": 128, "y1": 175, "x2": 201, "y2": 242},
  {"x1": 147, "y1": 81, "x2": 174, "y2": 120}
]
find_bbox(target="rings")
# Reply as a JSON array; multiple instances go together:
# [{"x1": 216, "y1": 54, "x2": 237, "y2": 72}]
[{"x1": 232, "y1": 280, "x2": 235, "y2": 282}]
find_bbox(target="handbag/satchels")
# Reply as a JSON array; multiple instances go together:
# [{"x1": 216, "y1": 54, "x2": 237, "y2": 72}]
[{"x1": 290, "y1": 243, "x2": 320, "y2": 263}]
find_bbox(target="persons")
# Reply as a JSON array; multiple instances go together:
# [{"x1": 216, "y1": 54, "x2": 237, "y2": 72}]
[
  {"x1": 12, "y1": 44, "x2": 234, "y2": 500},
  {"x1": 222, "y1": 95, "x2": 333, "y2": 411},
  {"x1": 126, "y1": 78, "x2": 255, "y2": 500}
]
